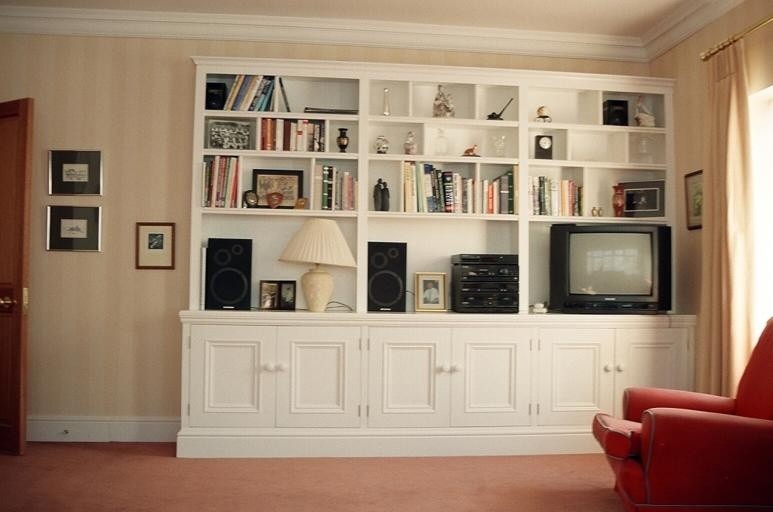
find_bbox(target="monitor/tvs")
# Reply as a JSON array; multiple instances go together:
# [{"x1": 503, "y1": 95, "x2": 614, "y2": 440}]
[{"x1": 547, "y1": 224, "x2": 673, "y2": 317}]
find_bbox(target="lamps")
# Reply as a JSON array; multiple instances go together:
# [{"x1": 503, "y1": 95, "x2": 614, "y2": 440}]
[{"x1": 278, "y1": 218, "x2": 358, "y2": 312}]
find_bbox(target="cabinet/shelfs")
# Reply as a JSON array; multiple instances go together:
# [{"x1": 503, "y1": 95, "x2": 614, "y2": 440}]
[{"x1": 175, "y1": 54, "x2": 698, "y2": 459}]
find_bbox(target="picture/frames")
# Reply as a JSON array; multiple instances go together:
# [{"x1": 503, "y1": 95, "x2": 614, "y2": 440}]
[
  {"x1": 413, "y1": 271, "x2": 448, "y2": 313},
  {"x1": 135, "y1": 221, "x2": 176, "y2": 270},
  {"x1": 683, "y1": 169, "x2": 706, "y2": 231}
]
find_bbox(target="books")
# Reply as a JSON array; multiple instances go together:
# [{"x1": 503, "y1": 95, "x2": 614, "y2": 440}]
[
  {"x1": 532, "y1": 174, "x2": 586, "y2": 217},
  {"x1": 314, "y1": 164, "x2": 358, "y2": 210},
  {"x1": 223, "y1": 75, "x2": 291, "y2": 114},
  {"x1": 204, "y1": 154, "x2": 238, "y2": 208},
  {"x1": 404, "y1": 162, "x2": 517, "y2": 214},
  {"x1": 260, "y1": 118, "x2": 325, "y2": 153}
]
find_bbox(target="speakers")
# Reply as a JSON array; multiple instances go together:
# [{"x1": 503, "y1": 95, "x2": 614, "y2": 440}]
[
  {"x1": 205, "y1": 238, "x2": 252, "y2": 310},
  {"x1": 367, "y1": 242, "x2": 407, "y2": 312}
]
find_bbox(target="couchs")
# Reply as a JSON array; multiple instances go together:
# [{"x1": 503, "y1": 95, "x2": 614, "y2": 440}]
[{"x1": 591, "y1": 317, "x2": 773, "y2": 512}]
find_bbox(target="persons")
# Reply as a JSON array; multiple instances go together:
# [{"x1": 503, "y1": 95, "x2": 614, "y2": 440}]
[
  {"x1": 423, "y1": 282, "x2": 439, "y2": 303},
  {"x1": 285, "y1": 287, "x2": 294, "y2": 307}
]
